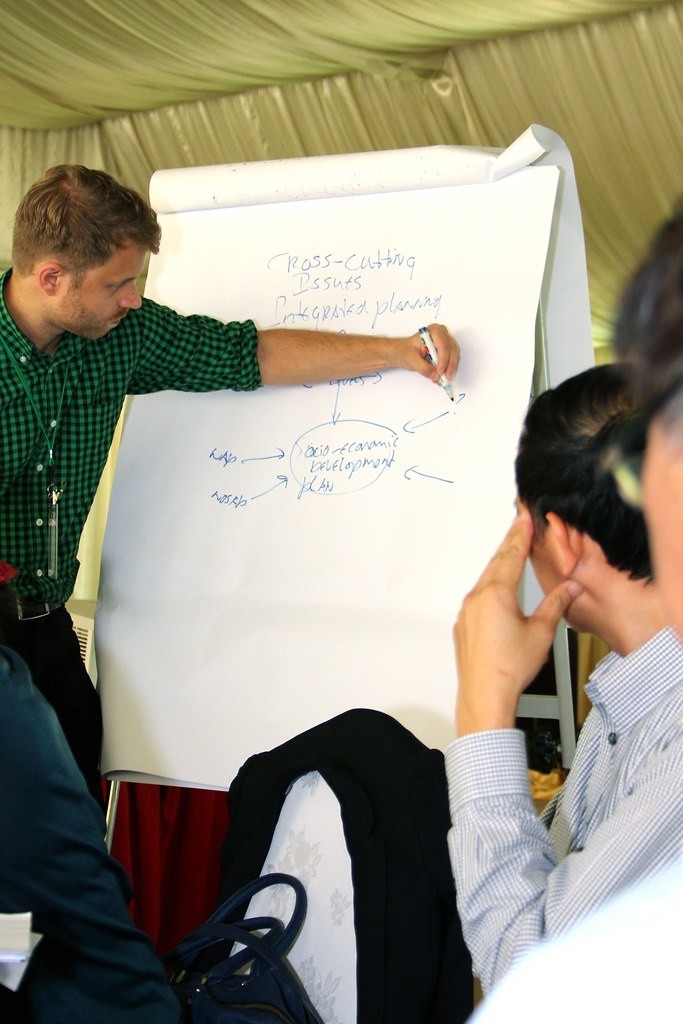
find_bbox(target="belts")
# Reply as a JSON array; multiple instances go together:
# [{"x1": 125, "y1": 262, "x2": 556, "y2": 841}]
[{"x1": 0, "y1": 599, "x2": 62, "y2": 620}]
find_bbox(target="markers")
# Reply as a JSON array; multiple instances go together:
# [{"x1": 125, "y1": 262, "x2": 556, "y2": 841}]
[{"x1": 419, "y1": 326, "x2": 454, "y2": 403}]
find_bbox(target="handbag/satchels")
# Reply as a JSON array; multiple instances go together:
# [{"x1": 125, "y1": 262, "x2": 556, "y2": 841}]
[{"x1": 165, "y1": 871, "x2": 307, "y2": 1024}]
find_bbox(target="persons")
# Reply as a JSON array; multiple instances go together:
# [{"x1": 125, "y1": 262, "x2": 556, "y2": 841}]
[
  {"x1": 2, "y1": 639, "x2": 181, "y2": 1024},
  {"x1": 455, "y1": 360, "x2": 683, "y2": 1000},
  {"x1": 457, "y1": 194, "x2": 683, "y2": 1024},
  {"x1": 0, "y1": 162, "x2": 461, "y2": 825}
]
